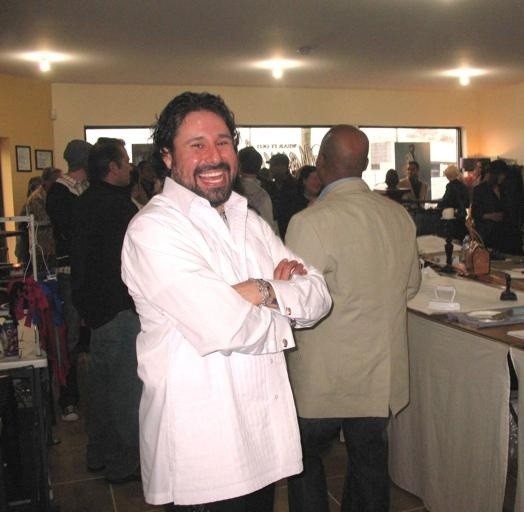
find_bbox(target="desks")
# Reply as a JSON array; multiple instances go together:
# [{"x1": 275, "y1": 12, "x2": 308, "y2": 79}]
[{"x1": 404, "y1": 236, "x2": 524, "y2": 512}]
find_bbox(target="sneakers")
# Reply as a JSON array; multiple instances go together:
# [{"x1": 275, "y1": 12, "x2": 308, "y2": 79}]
[
  {"x1": 60, "y1": 405, "x2": 79, "y2": 421},
  {"x1": 87, "y1": 459, "x2": 106, "y2": 473},
  {"x1": 106, "y1": 461, "x2": 141, "y2": 483}
]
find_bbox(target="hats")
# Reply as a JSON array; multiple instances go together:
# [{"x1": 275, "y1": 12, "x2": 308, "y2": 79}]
[
  {"x1": 266, "y1": 154, "x2": 289, "y2": 165},
  {"x1": 64, "y1": 139, "x2": 92, "y2": 171}
]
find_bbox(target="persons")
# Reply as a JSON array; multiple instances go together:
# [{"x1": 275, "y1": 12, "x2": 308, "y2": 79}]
[
  {"x1": 384, "y1": 156, "x2": 523, "y2": 258},
  {"x1": 13, "y1": 137, "x2": 170, "y2": 485},
  {"x1": 284, "y1": 124, "x2": 420, "y2": 511},
  {"x1": 119, "y1": 92, "x2": 333, "y2": 511},
  {"x1": 403, "y1": 143, "x2": 417, "y2": 162},
  {"x1": 231, "y1": 144, "x2": 325, "y2": 241}
]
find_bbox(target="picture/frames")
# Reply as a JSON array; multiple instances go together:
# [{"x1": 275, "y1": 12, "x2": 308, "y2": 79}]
[{"x1": 14, "y1": 145, "x2": 52, "y2": 173}]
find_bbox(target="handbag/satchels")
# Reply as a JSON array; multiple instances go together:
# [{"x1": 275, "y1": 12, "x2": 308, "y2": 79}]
[{"x1": 460, "y1": 240, "x2": 489, "y2": 275}]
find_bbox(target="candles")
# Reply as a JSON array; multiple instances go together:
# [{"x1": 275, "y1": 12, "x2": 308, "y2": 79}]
[{"x1": 441, "y1": 208, "x2": 454, "y2": 218}]
